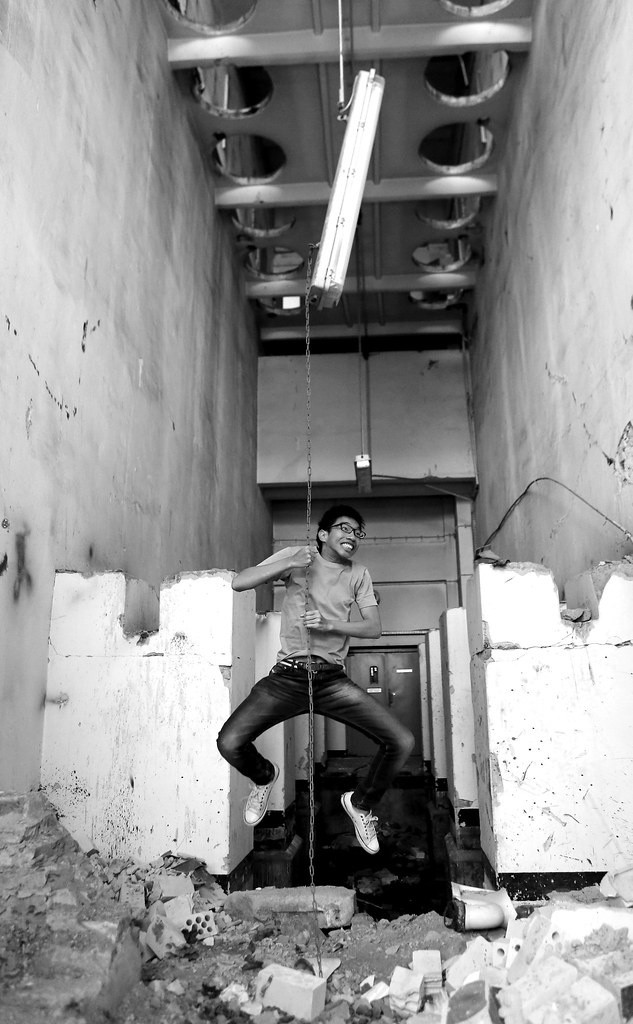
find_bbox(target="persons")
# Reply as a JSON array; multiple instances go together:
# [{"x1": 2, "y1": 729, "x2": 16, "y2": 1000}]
[{"x1": 216, "y1": 505, "x2": 416, "y2": 854}]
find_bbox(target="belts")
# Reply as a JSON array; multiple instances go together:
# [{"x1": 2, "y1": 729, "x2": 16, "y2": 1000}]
[{"x1": 280, "y1": 659, "x2": 343, "y2": 671}]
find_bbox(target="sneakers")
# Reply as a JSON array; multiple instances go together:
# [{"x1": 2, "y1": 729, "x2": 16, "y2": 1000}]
[
  {"x1": 341, "y1": 791, "x2": 379, "y2": 855},
  {"x1": 243, "y1": 763, "x2": 280, "y2": 826}
]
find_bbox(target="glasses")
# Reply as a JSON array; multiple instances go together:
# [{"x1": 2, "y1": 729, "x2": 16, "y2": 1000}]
[{"x1": 329, "y1": 522, "x2": 366, "y2": 540}]
